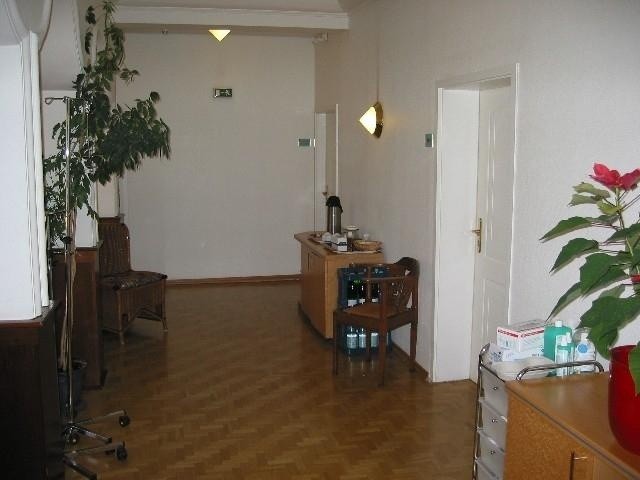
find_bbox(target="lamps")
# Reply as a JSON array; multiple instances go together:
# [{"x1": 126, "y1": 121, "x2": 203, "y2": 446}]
[
  {"x1": 358, "y1": 101, "x2": 383, "y2": 138},
  {"x1": 209, "y1": 30, "x2": 231, "y2": 43}
]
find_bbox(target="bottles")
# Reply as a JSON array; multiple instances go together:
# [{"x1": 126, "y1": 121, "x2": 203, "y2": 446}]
[
  {"x1": 326, "y1": 196, "x2": 343, "y2": 235},
  {"x1": 543, "y1": 320, "x2": 597, "y2": 377},
  {"x1": 345, "y1": 261, "x2": 391, "y2": 356}
]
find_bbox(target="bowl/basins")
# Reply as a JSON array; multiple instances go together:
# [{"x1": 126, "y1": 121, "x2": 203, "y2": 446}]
[{"x1": 355, "y1": 241, "x2": 381, "y2": 251}]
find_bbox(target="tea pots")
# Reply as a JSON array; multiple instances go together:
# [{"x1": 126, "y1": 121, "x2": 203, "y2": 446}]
[{"x1": 344, "y1": 224, "x2": 359, "y2": 252}]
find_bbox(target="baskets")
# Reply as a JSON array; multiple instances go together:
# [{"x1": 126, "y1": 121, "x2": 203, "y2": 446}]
[{"x1": 354, "y1": 240, "x2": 381, "y2": 251}]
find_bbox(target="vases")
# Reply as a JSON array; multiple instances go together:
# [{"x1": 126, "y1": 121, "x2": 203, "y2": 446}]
[{"x1": 607, "y1": 344, "x2": 640, "y2": 456}]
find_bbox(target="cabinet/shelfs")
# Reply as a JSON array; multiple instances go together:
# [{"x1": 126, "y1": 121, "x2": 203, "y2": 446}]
[
  {"x1": 294, "y1": 231, "x2": 384, "y2": 341},
  {"x1": 472, "y1": 344, "x2": 604, "y2": 480},
  {"x1": 53, "y1": 247, "x2": 106, "y2": 390},
  {"x1": 505, "y1": 371, "x2": 640, "y2": 480}
]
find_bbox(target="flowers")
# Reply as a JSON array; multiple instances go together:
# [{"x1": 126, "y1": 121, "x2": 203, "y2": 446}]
[{"x1": 536, "y1": 163, "x2": 640, "y2": 398}]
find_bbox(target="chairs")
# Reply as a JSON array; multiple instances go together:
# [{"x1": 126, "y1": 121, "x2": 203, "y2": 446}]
[
  {"x1": 334, "y1": 257, "x2": 418, "y2": 385},
  {"x1": 97, "y1": 216, "x2": 168, "y2": 345}
]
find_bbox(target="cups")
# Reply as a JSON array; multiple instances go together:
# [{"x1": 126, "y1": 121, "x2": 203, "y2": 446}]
[{"x1": 322, "y1": 232, "x2": 342, "y2": 244}]
